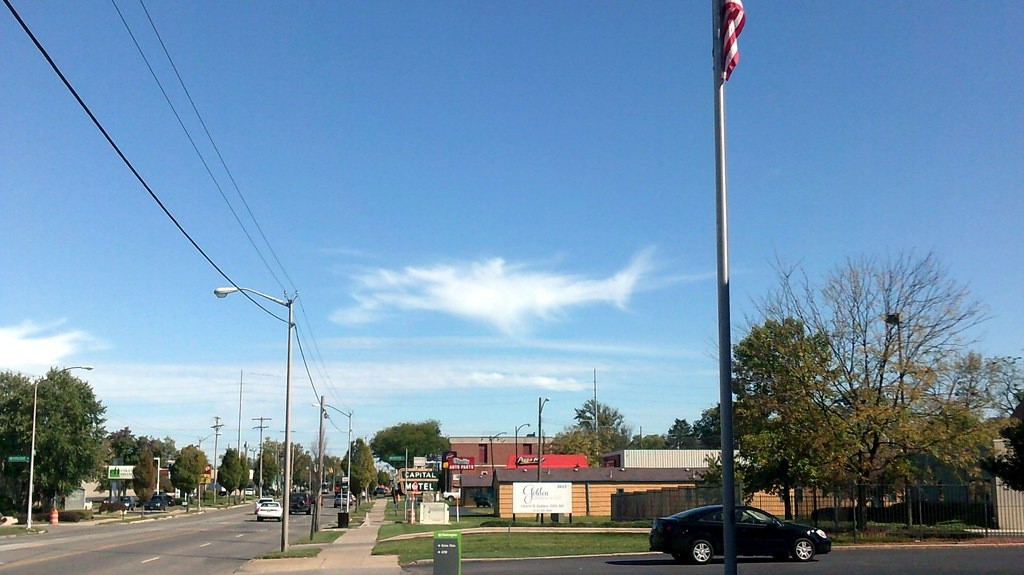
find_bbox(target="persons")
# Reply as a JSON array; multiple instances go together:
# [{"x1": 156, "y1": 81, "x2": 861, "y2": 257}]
[
  {"x1": 713, "y1": 513, "x2": 722, "y2": 520},
  {"x1": 735, "y1": 511, "x2": 750, "y2": 523}
]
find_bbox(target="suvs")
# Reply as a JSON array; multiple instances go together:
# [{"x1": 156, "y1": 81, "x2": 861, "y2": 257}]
[{"x1": 289, "y1": 493, "x2": 323, "y2": 515}]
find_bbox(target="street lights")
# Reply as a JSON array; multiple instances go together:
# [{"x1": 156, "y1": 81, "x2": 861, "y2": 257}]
[
  {"x1": 489, "y1": 431, "x2": 507, "y2": 471},
  {"x1": 515, "y1": 423, "x2": 530, "y2": 469},
  {"x1": 27, "y1": 366, "x2": 94, "y2": 530},
  {"x1": 536, "y1": 397, "x2": 549, "y2": 522},
  {"x1": 214, "y1": 287, "x2": 292, "y2": 552},
  {"x1": 884, "y1": 311, "x2": 912, "y2": 529}
]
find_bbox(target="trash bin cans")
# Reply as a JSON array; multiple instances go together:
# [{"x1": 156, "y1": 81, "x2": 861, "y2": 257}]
[{"x1": 338, "y1": 512, "x2": 348, "y2": 527}]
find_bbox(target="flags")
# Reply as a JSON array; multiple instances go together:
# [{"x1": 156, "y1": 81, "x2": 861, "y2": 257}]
[{"x1": 712, "y1": 0, "x2": 746, "y2": 81}]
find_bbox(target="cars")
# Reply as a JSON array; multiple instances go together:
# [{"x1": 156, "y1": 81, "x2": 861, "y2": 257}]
[
  {"x1": 99, "y1": 496, "x2": 135, "y2": 514},
  {"x1": 245, "y1": 488, "x2": 256, "y2": 496},
  {"x1": 254, "y1": 498, "x2": 283, "y2": 522},
  {"x1": 334, "y1": 494, "x2": 356, "y2": 508},
  {"x1": 649, "y1": 505, "x2": 832, "y2": 565},
  {"x1": 145, "y1": 491, "x2": 176, "y2": 511},
  {"x1": 312, "y1": 403, "x2": 352, "y2": 513}
]
